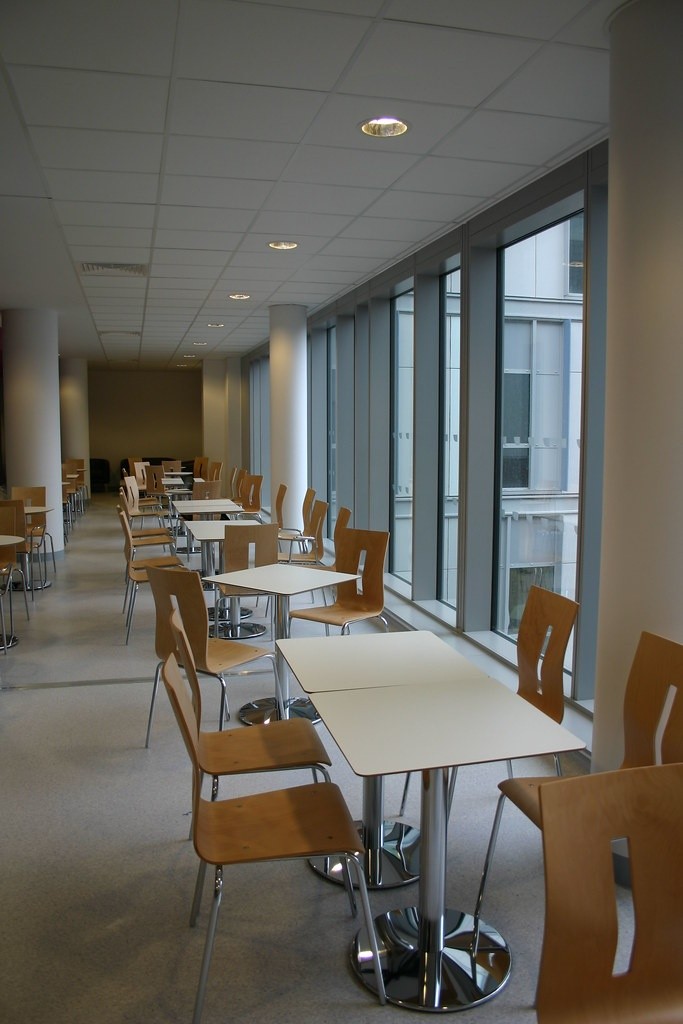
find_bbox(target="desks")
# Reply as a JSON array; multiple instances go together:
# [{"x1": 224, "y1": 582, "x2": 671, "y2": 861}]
[
  {"x1": 277, "y1": 630, "x2": 489, "y2": 887},
  {"x1": 171, "y1": 499, "x2": 235, "y2": 579},
  {"x1": 162, "y1": 472, "x2": 193, "y2": 508},
  {"x1": 161, "y1": 480, "x2": 184, "y2": 520},
  {"x1": 306, "y1": 678, "x2": 586, "y2": 1015},
  {"x1": 0, "y1": 507, "x2": 55, "y2": 591},
  {"x1": 160, "y1": 477, "x2": 182, "y2": 516},
  {"x1": 190, "y1": 527, "x2": 282, "y2": 640},
  {"x1": 201, "y1": 564, "x2": 363, "y2": 727},
  {"x1": 184, "y1": 520, "x2": 262, "y2": 621},
  {"x1": 0, "y1": 535, "x2": 25, "y2": 650},
  {"x1": 176, "y1": 505, "x2": 245, "y2": 591}
]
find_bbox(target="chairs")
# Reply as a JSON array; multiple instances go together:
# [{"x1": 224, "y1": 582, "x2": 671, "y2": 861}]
[{"x1": 0, "y1": 455, "x2": 683, "y2": 1024}]
